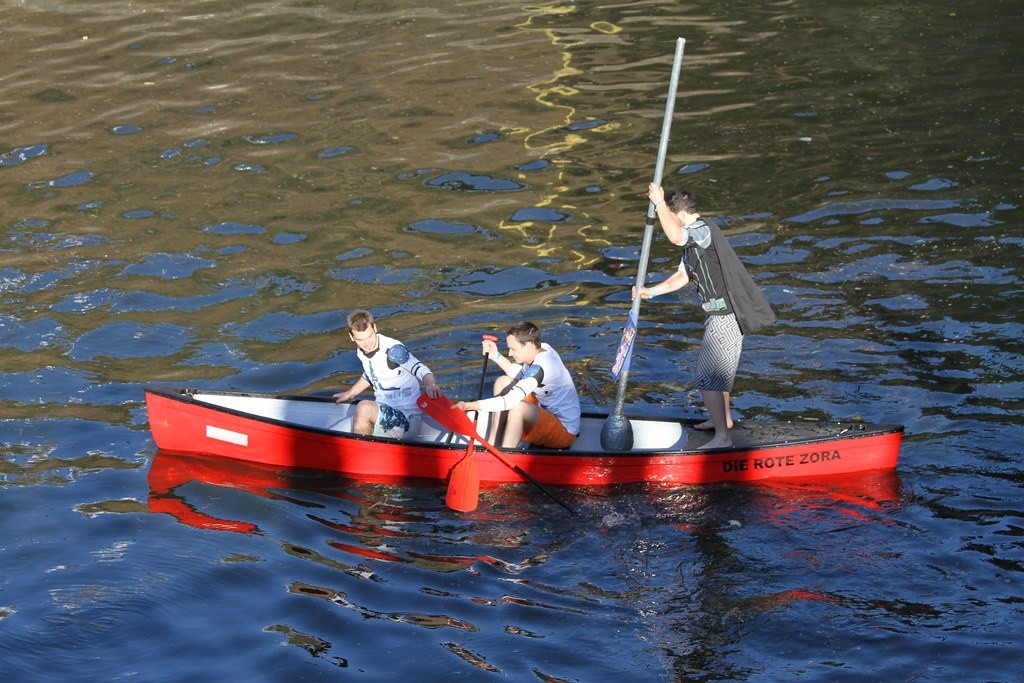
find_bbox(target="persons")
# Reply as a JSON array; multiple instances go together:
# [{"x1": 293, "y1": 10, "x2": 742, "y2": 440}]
[
  {"x1": 631, "y1": 182, "x2": 744, "y2": 450},
  {"x1": 332, "y1": 308, "x2": 442, "y2": 440},
  {"x1": 452, "y1": 321, "x2": 581, "y2": 450}
]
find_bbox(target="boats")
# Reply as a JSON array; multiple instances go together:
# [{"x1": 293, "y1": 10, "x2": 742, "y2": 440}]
[{"x1": 143, "y1": 386, "x2": 904, "y2": 485}]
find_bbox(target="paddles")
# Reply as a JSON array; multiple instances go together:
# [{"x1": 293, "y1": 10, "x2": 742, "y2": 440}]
[
  {"x1": 415, "y1": 388, "x2": 570, "y2": 512},
  {"x1": 444, "y1": 332, "x2": 499, "y2": 511}
]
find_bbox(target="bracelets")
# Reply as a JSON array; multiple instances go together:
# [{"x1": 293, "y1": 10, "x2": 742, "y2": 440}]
[
  {"x1": 652, "y1": 287, "x2": 656, "y2": 297},
  {"x1": 492, "y1": 352, "x2": 501, "y2": 362},
  {"x1": 656, "y1": 200, "x2": 665, "y2": 206}
]
